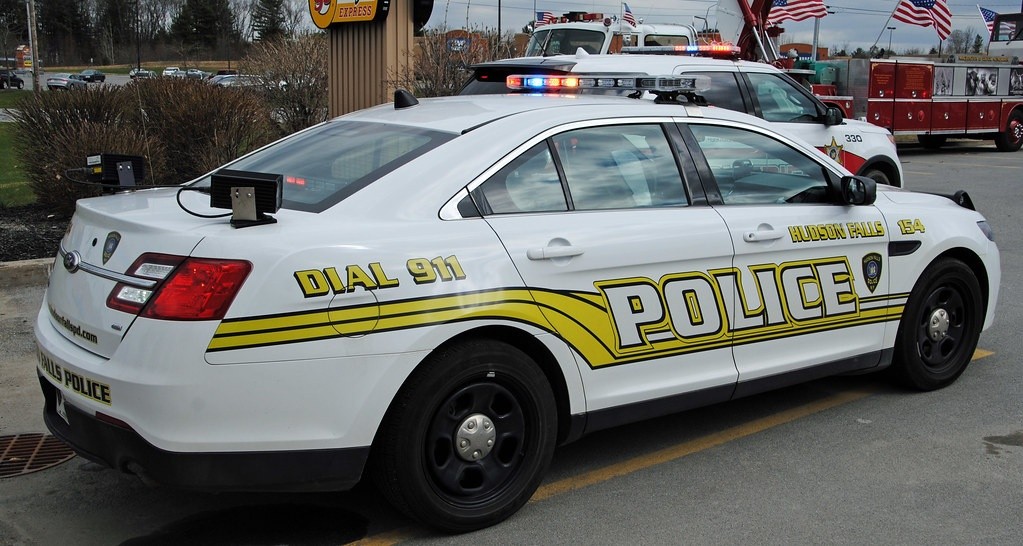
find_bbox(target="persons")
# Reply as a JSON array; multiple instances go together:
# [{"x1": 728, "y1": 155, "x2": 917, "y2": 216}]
[
  {"x1": 940, "y1": 71, "x2": 950, "y2": 94},
  {"x1": 1011, "y1": 71, "x2": 1023, "y2": 91},
  {"x1": 969, "y1": 70, "x2": 996, "y2": 96}
]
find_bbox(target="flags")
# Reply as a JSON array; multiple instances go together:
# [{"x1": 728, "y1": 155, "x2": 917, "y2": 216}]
[
  {"x1": 536, "y1": 12, "x2": 554, "y2": 24},
  {"x1": 980, "y1": 6, "x2": 1016, "y2": 35},
  {"x1": 766, "y1": 0, "x2": 827, "y2": 25},
  {"x1": 623, "y1": 3, "x2": 637, "y2": 28},
  {"x1": 892, "y1": 0, "x2": 952, "y2": 41}
]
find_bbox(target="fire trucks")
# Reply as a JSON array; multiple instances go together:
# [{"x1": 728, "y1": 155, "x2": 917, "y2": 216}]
[{"x1": 523, "y1": 0, "x2": 1023, "y2": 151}]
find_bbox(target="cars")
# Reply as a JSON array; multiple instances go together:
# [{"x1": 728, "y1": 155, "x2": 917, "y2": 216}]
[
  {"x1": 47, "y1": 72, "x2": 89, "y2": 93},
  {"x1": 129, "y1": 64, "x2": 291, "y2": 92},
  {"x1": 0, "y1": 67, "x2": 25, "y2": 89},
  {"x1": 31, "y1": 72, "x2": 1002, "y2": 532},
  {"x1": 78, "y1": 69, "x2": 107, "y2": 83}
]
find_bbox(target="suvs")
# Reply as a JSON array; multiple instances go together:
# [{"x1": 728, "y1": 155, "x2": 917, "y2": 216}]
[{"x1": 451, "y1": 43, "x2": 906, "y2": 191}]
